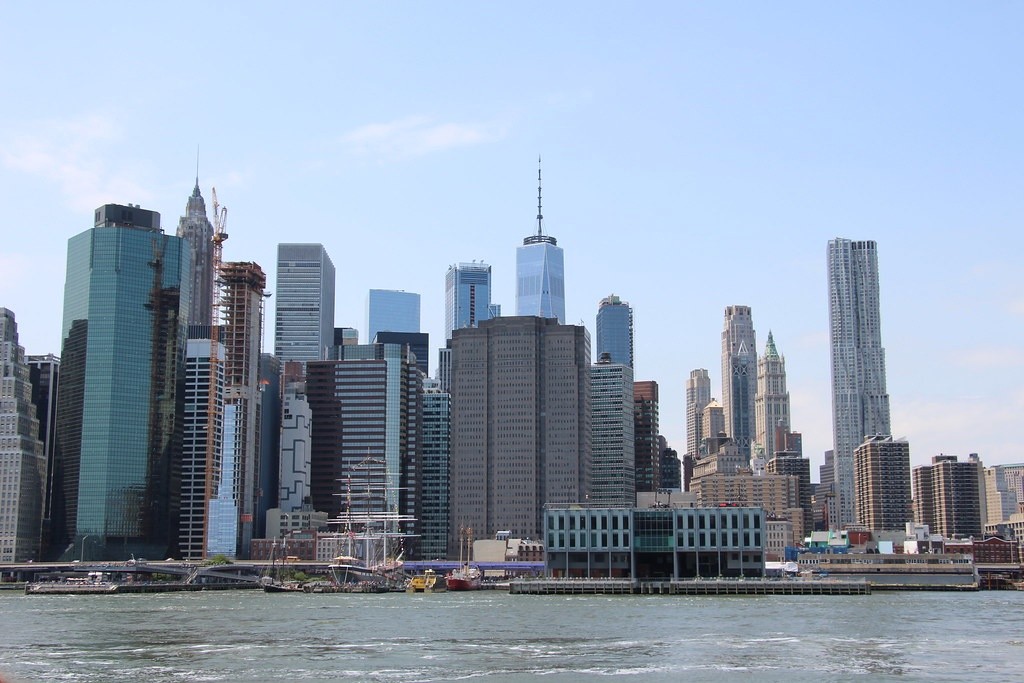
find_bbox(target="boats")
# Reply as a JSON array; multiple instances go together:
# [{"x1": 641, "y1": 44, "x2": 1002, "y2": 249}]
[
  {"x1": 443, "y1": 520, "x2": 486, "y2": 588},
  {"x1": 24, "y1": 573, "x2": 120, "y2": 595},
  {"x1": 327, "y1": 448, "x2": 410, "y2": 593},
  {"x1": 254, "y1": 536, "x2": 306, "y2": 592},
  {"x1": 408, "y1": 568, "x2": 438, "y2": 592}
]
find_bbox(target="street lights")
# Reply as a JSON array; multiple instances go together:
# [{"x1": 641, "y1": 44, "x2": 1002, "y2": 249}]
[{"x1": 79, "y1": 535, "x2": 89, "y2": 564}]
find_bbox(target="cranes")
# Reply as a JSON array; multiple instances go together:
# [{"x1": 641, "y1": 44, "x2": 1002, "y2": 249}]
[{"x1": 200, "y1": 187, "x2": 234, "y2": 561}]
